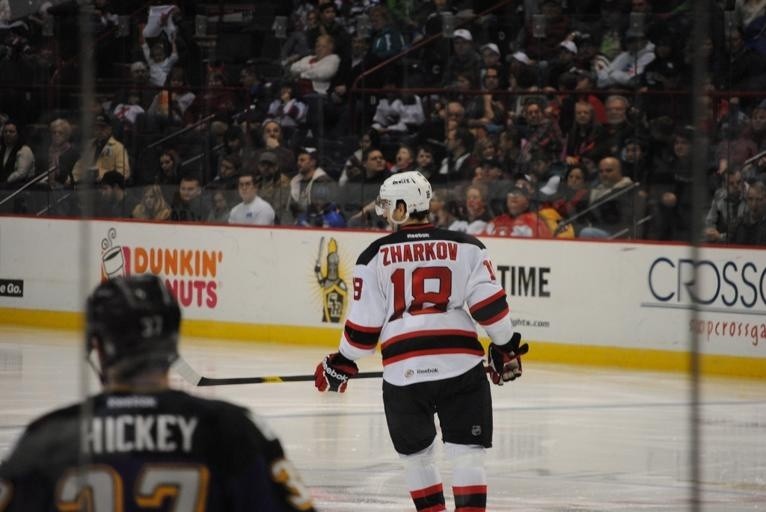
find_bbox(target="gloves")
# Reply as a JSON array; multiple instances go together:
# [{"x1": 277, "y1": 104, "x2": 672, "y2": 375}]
[
  {"x1": 488, "y1": 334, "x2": 529, "y2": 385},
  {"x1": 315, "y1": 354, "x2": 357, "y2": 393}
]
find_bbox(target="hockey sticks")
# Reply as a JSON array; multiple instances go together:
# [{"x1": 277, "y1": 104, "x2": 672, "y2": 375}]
[{"x1": 173, "y1": 356, "x2": 386, "y2": 387}]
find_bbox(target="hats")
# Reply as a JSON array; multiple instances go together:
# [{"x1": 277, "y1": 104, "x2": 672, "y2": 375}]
[
  {"x1": 506, "y1": 51, "x2": 529, "y2": 66},
  {"x1": 539, "y1": 175, "x2": 566, "y2": 197},
  {"x1": 555, "y1": 40, "x2": 577, "y2": 55},
  {"x1": 509, "y1": 184, "x2": 527, "y2": 197},
  {"x1": 451, "y1": 29, "x2": 472, "y2": 42},
  {"x1": 480, "y1": 41, "x2": 500, "y2": 56}
]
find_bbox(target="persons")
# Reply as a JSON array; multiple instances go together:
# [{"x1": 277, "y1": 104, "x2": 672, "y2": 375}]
[
  {"x1": 0, "y1": 274, "x2": 315, "y2": 511},
  {"x1": 315, "y1": 176, "x2": 524, "y2": 511},
  {"x1": 1, "y1": 1, "x2": 766, "y2": 253}
]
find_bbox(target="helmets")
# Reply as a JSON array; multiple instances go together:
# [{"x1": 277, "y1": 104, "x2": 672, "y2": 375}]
[
  {"x1": 374, "y1": 171, "x2": 433, "y2": 224},
  {"x1": 83, "y1": 273, "x2": 180, "y2": 388},
  {"x1": 293, "y1": 145, "x2": 320, "y2": 162}
]
